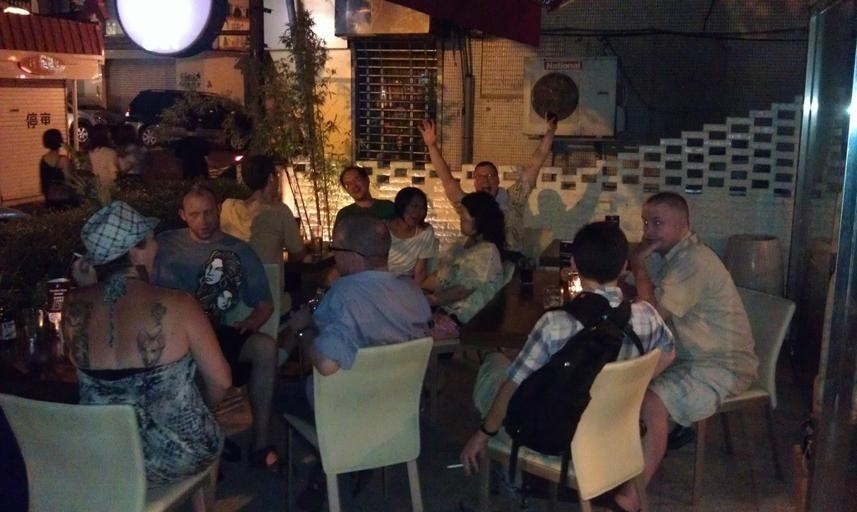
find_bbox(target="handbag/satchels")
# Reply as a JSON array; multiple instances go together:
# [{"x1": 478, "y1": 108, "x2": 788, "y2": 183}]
[{"x1": 45, "y1": 183, "x2": 78, "y2": 207}]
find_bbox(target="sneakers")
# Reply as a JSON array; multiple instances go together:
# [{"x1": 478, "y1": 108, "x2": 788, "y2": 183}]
[{"x1": 667, "y1": 423, "x2": 695, "y2": 449}]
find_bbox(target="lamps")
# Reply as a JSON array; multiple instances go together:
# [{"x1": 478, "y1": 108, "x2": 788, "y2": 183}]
[{"x1": 113, "y1": 0, "x2": 229, "y2": 57}]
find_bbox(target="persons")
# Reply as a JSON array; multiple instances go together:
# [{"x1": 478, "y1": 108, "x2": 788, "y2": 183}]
[
  {"x1": 33, "y1": 126, "x2": 439, "y2": 512},
  {"x1": 458, "y1": 220, "x2": 677, "y2": 512},
  {"x1": 611, "y1": 192, "x2": 762, "y2": 512},
  {"x1": 417, "y1": 111, "x2": 557, "y2": 340},
  {"x1": 419, "y1": 184, "x2": 507, "y2": 402}
]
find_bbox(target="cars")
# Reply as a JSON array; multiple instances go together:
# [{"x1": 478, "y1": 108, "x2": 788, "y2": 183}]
[{"x1": 67, "y1": 102, "x2": 123, "y2": 147}]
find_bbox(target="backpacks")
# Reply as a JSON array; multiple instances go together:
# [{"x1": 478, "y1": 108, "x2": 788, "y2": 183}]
[{"x1": 503, "y1": 300, "x2": 631, "y2": 455}]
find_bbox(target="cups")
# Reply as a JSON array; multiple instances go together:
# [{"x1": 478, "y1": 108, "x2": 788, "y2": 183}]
[
  {"x1": 542, "y1": 285, "x2": 563, "y2": 310},
  {"x1": 518, "y1": 257, "x2": 535, "y2": 285},
  {"x1": 309, "y1": 225, "x2": 323, "y2": 256},
  {"x1": 0, "y1": 298, "x2": 65, "y2": 371},
  {"x1": 567, "y1": 271, "x2": 583, "y2": 301}
]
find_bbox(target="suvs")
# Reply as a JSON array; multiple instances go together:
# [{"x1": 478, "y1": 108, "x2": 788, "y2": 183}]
[{"x1": 123, "y1": 89, "x2": 255, "y2": 150}]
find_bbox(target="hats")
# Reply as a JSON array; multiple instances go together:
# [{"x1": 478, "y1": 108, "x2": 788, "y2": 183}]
[{"x1": 80, "y1": 200, "x2": 161, "y2": 265}]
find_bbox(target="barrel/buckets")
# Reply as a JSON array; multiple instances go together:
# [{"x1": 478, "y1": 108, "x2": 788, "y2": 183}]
[{"x1": 724, "y1": 235, "x2": 784, "y2": 297}]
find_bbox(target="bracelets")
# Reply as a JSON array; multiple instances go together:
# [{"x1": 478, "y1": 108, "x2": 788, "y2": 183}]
[{"x1": 479, "y1": 423, "x2": 499, "y2": 438}]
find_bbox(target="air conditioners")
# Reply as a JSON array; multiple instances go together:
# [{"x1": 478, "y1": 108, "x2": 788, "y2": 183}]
[{"x1": 521, "y1": 55, "x2": 624, "y2": 142}]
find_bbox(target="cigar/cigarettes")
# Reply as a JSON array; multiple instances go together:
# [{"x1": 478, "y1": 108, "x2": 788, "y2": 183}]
[{"x1": 446, "y1": 462, "x2": 466, "y2": 471}]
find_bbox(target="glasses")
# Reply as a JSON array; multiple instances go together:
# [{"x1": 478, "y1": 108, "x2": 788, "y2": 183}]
[{"x1": 327, "y1": 243, "x2": 365, "y2": 257}]
